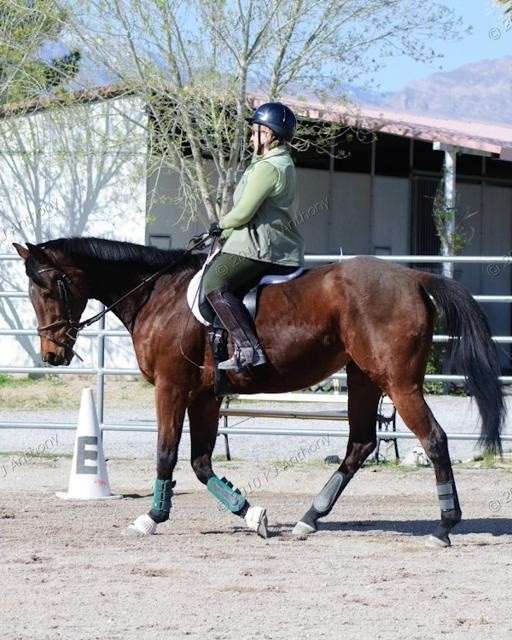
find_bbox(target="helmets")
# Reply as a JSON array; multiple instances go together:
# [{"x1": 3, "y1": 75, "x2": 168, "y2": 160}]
[{"x1": 245, "y1": 102, "x2": 296, "y2": 142}]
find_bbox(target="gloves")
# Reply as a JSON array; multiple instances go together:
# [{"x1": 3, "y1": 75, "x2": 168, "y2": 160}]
[{"x1": 209, "y1": 223, "x2": 223, "y2": 236}]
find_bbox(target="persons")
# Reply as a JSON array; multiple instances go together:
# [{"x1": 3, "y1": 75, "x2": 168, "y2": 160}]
[{"x1": 206, "y1": 103, "x2": 305, "y2": 373}]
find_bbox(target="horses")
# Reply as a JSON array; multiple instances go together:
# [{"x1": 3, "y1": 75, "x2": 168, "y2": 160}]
[{"x1": 10, "y1": 235, "x2": 509, "y2": 548}]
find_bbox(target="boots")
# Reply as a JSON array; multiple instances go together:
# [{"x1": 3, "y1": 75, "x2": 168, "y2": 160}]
[{"x1": 206, "y1": 285, "x2": 268, "y2": 370}]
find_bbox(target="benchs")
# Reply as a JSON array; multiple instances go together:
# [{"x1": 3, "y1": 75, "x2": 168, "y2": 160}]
[{"x1": 224, "y1": 367, "x2": 400, "y2": 464}]
